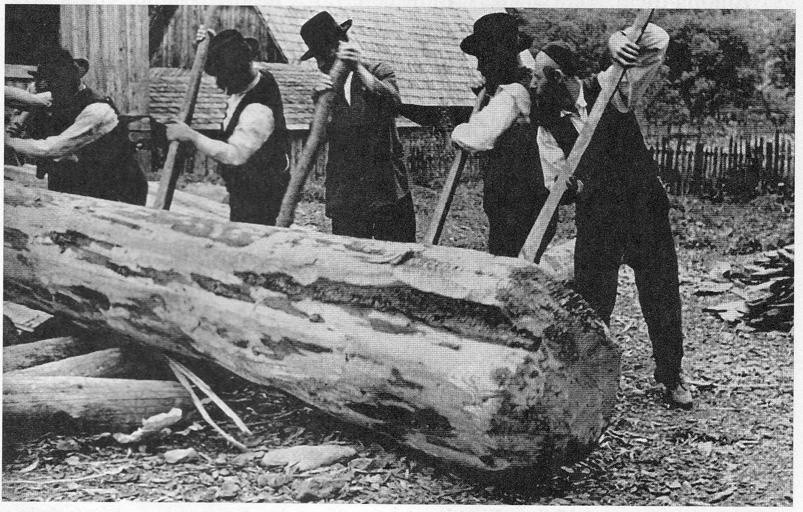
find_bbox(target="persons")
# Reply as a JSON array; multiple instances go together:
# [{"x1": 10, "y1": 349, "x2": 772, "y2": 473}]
[
  {"x1": 5, "y1": 47, "x2": 149, "y2": 207},
  {"x1": 450, "y1": 12, "x2": 558, "y2": 263},
  {"x1": 298, "y1": 11, "x2": 419, "y2": 243},
  {"x1": 164, "y1": 23, "x2": 292, "y2": 225},
  {"x1": 530, "y1": 22, "x2": 695, "y2": 409}
]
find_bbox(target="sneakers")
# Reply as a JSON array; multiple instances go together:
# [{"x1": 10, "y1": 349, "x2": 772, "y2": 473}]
[{"x1": 654, "y1": 373, "x2": 692, "y2": 408}]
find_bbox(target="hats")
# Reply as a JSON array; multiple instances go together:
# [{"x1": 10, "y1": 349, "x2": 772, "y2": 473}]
[
  {"x1": 205, "y1": 29, "x2": 259, "y2": 76},
  {"x1": 28, "y1": 50, "x2": 89, "y2": 81},
  {"x1": 460, "y1": 13, "x2": 532, "y2": 55},
  {"x1": 301, "y1": 11, "x2": 352, "y2": 61}
]
